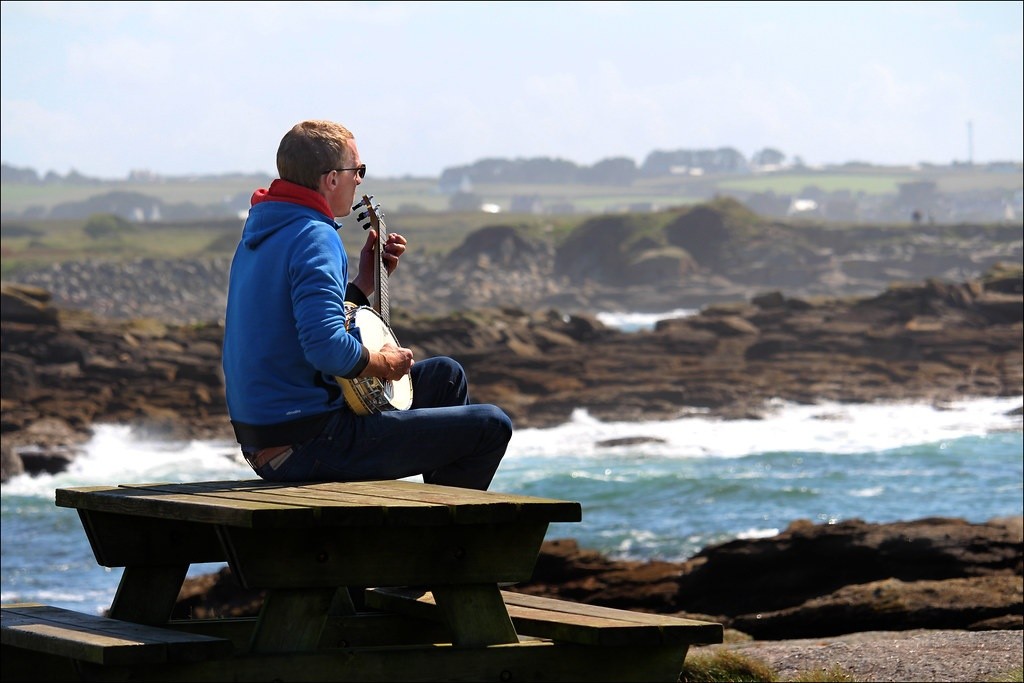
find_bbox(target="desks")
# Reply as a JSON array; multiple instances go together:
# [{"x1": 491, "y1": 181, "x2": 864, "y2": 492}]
[{"x1": 77, "y1": 479, "x2": 582, "y2": 683}]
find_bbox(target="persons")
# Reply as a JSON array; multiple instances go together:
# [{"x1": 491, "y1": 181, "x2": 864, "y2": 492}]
[{"x1": 220, "y1": 121, "x2": 515, "y2": 494}]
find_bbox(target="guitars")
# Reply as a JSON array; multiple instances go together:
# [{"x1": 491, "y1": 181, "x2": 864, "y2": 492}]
[{"x1": 334, "y1": 193, "x2": 414, "y2": 415}]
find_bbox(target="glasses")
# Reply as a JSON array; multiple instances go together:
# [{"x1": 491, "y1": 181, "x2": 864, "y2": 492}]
[{"x1": 320, "y1": 164, "x2": 366, "y2": 179}]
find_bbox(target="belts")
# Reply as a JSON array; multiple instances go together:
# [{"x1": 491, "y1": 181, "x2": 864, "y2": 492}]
[{"x1": 252, "y1": 445, "x2": 292, "y2": 468}]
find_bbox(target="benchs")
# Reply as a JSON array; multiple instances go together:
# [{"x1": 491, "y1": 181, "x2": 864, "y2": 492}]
[
  {"x1": 364, "y1": 586, "x2": 725, "y2": 683},
  {"x1": 1, "y1": 605, "x2": 234, "y2": 683}
]
